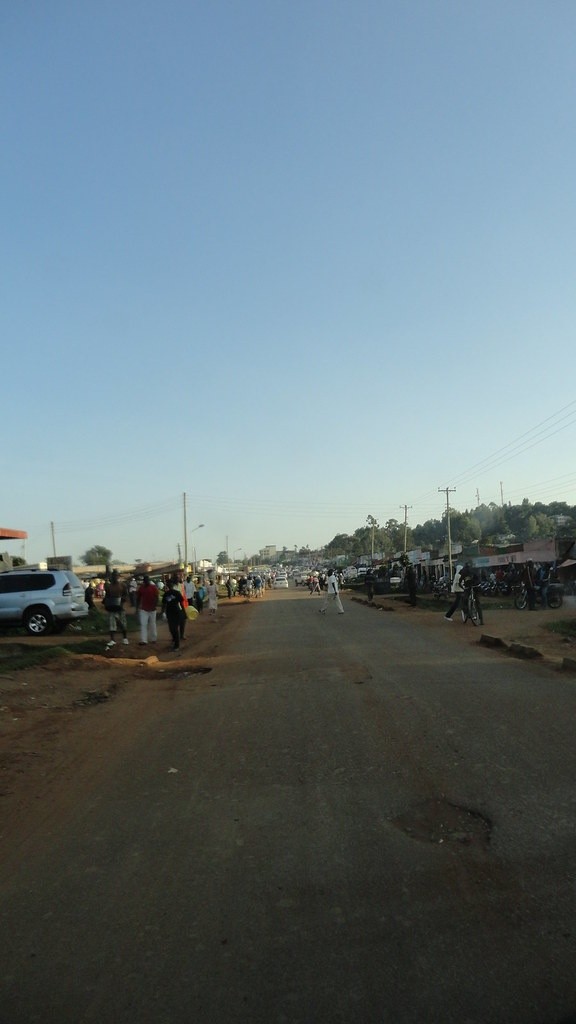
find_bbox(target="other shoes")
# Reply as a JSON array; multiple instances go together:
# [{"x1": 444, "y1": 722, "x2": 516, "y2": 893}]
[
  {"x1": 180, "y1": 637, "x2": 187, "y2": 640},
  {"x1": 107, "y1": 640, "x2": 117, "y2": 646},
  {"x1": 122, "y1": 638, "x2": 129, "y2": 645},
  {"x1": 151, "y1": 641, "x2": 160, "y2": 644},
  {"x1": 529, "y1": 608, "x2": 538, "y2": 611},
  {"x1": 444, "y1": 615, "x2": 453, "y2": 621},
  {"x1": 174, "y1": 648, "x2": 179, "y2": 652},
  {"x1": 472, "y1": 616, "x2": 477, "y2": 619},
  {"x1": 319, "y1": 609, "x2": 327, "y2": 614},
  {"x1": 336, "y1": 612, "x2": 345, "y2": 614},
  {"x1": 139, "y1": 640, "x2": 148, "y2": 645}
]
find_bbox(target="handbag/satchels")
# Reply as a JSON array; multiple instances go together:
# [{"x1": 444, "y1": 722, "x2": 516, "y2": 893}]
[{"x1": 531, "y1": 585, "x2": 540, "y2": 590}]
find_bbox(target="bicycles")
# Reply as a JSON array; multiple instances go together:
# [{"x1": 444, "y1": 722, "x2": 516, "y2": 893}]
[{"x1": 460, "y1": 580, "x2": 482, "y2": 626}]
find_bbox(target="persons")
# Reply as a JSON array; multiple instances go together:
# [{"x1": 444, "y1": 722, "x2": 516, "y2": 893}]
[
  {"x1": 135, "y1": 574, "x2": 159, "y2": 645},
  {"x1": 161, "y1": 579, "x2": 189, "y2": 652},
  {"x1": 490, "y1": 558, "x2": 553, "y2": 611},
  {"x1": 437, "y1": 561, "x2": 485, "y2": 627},
  {"x1": 103, "y1": 569, "x2": 129, "y2": 647},
  {"x1": 406, "y1": 564, "x2": 417, "y2": 607},
  {"x1": 302, "y1": 557, "x2": 350, "y2": 615},
  {"x1": 363, "y1": 568, "x2": 376, "y2": 601},
  {"x1": 85, "y1": 565, "x2": 279, "y2": 619}
]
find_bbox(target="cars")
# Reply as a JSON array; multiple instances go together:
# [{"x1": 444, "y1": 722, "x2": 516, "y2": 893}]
[
  {"x1": 273, "y1": 577, "x2": 288, "y2": 588},
  {"x1": 278, "y1": 572, "x2": 288, "y2": 579},
  {"x1": 290, "y1": 569, "x2": 312, "y2": 586}
]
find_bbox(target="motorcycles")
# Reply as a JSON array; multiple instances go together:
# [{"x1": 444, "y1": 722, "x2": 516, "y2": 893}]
[
  {"x1": 479, "y1": 578, "x2": 511, "y2": 597},
  {"x1": 514, "y1": 579, "x2": 565, "y2": 609}
]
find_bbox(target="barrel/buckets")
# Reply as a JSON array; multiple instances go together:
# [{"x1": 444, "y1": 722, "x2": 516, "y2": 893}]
[{"x1": 185, "y1": 605, "x2": 199, "y2": 620}]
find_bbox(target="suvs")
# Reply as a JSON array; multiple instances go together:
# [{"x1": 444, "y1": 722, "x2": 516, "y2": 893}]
[{"x1": 0, "y1": 569, "x2": 89, "y2": 635}]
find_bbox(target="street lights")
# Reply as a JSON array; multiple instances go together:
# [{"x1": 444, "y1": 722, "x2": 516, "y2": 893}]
[
  {"x1": 184, "y1": 524, "x2": 205, "y2": 581},
  {"x1": 227, "y1": 548, "x2": 242, "y2": 579}
]
